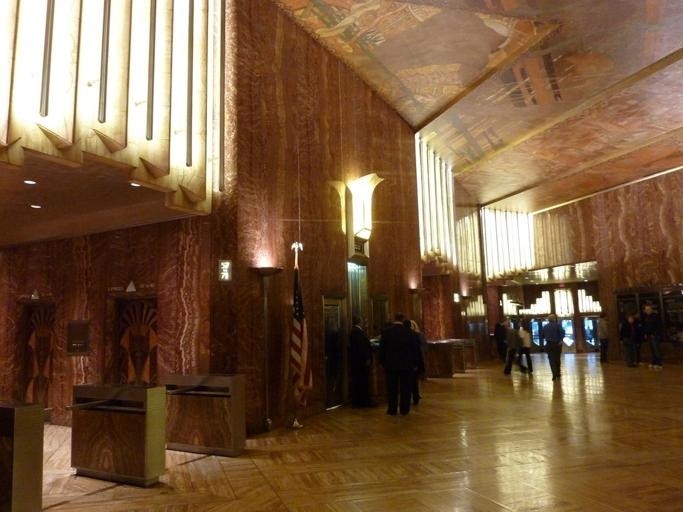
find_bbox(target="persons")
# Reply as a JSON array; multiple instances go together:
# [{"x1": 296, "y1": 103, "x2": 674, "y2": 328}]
[
  {"x1": 494, "y1": 317, "x2": 534, "y2": 376},
  {"x1": 347, "y1": 311, "x2": 428, "y2": 416},
  {"x1": 641, "y1": 304, "x2": 664, "y2": 369},
  {"x1": 586, "y1": 326, "x2": 593, "y2": 343},
  {"x1": 618, "y1": 311, "x2": 637, "y2": 367},
  {"x1": 541, "y1": 313, "x2": 566, "y2": 381},
  {"x1": 596, "y1": 311, "x2": 611, "y2": 363},
  {"x1": 324, "y1": 315, "x2": 342, "y2": 390},
  {"x1": 627, "y1": 315, "x2": 644, "y2": 366}
]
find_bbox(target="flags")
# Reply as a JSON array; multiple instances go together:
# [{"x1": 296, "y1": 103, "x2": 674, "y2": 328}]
[{"x1": 290, "y1": 268, "x2": 313, "y2": 407}]
